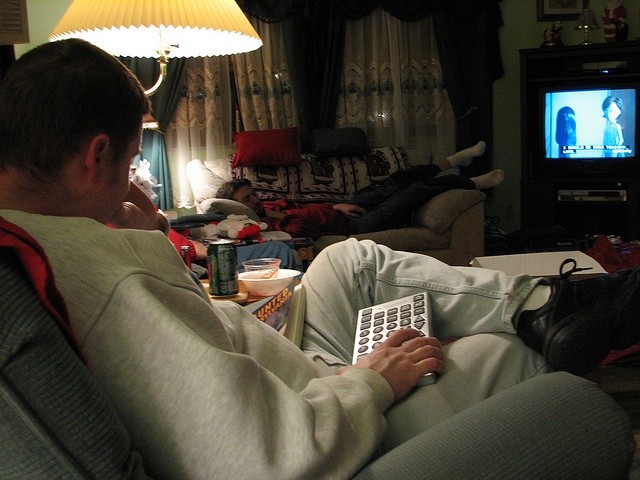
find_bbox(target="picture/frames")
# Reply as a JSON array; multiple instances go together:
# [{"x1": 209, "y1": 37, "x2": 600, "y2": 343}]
[{"x1": 534, "y1": 0, "x2": 590, "y2": 22}]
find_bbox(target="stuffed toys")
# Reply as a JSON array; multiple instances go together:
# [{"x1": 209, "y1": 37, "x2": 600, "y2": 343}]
[
  {"x1": 551, "y1": 19, "x2": 564, "y2": 43},
  {"x1": 601, "y1": 1, "x2": 628, "y2": 42}
]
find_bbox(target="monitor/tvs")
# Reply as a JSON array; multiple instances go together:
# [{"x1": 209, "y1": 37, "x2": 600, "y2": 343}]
[{"x1": 538, "y1": 84, "x2": 640, "y2": 176}]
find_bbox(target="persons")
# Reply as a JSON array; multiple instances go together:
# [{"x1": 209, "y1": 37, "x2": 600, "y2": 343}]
[
  {"x1": 0, "y1": 38, "x2": 639, "y2": 479},
  {"x1": 106, "y1": 203, "x2": 296, "y2": 278},
  {"x1": 601, "y1": 95, "x2": 625, "y2": 158},
  {"x1": 214, "y1": 141, "x2": 505, "y2": 239}
]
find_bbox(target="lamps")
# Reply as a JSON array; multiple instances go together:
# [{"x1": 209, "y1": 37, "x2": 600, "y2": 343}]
[
  {"x1": 573, "y1": 6, "x2": 600, "y2": 45},
  {"x1": 48, "y1": 0, "x2": 262, "y2": 129}
]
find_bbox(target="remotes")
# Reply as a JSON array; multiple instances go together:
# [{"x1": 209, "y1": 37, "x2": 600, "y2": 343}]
[{"x1": 351, "y1": 292, "x2": 437, "y2": 387}]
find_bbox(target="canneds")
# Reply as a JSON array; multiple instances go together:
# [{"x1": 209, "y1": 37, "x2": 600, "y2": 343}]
[{"x1": 207, "y1": 241, "x2": 239, "y2": 298}]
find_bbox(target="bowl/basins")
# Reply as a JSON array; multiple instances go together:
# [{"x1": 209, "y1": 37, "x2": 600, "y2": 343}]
[{"x1": 238, "y1": 269, "x2": 302, "y2": 297}]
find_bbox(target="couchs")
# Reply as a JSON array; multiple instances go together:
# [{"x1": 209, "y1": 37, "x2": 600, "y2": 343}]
[
  {"x1": 185, "y1": 125, "x2": 486, "y2": 277},
  {"x1": 0, "y1": 240, "x2": 636, "y2": 480}
]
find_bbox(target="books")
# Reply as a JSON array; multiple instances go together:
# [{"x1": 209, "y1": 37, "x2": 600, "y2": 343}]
[{"x1": 251, "y1": 277, "x2": 307, "y2": 349}]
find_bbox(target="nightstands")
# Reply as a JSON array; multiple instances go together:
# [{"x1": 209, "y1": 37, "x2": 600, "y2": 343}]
[{"x1": 192, "y1": 276, "x2": 306, "y2": 305}]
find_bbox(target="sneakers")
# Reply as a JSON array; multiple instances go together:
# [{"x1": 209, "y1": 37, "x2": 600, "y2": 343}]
[{"x1": 517, "y1": 258, "x2": 640, "y2": 371}]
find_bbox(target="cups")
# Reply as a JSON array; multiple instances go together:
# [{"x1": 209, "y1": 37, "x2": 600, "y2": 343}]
[{"x1": 241, "y1": 257, "x2": 281, "y2": 279}]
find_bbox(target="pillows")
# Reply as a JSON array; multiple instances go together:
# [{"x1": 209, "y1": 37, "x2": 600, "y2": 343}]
[
  {"x1": 232, "y1": 128, "x2": 305, "y2": 168},
  {"x1": 414, "y1": 188, "x2": 484, "y2": 232},
  {"x1": 301, "y1": 126, "x2": 370, "y2": 158},
  {"x1": 202, "y1": 196, "x2": 263, "y2": 228}
]
